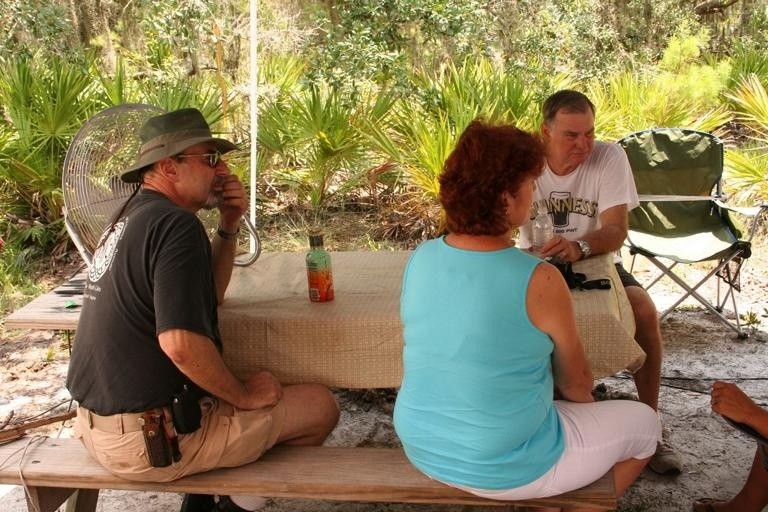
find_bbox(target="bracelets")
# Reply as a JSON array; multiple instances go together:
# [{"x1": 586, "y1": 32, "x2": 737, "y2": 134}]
[{"x1": 218, "y1": 228, "x2": 241, "y2": 239}]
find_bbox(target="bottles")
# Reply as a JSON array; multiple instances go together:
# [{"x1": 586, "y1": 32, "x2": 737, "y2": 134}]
[
  {"x1": 532, "y1": 204, "x2": 553, "y2": 265},
  {"x1": 304, "y1": 228, "x2": 336, "y2": 304}
]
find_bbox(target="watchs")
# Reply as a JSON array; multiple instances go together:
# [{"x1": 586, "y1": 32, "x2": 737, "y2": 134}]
[{"x1": 574, "y1": 239, "x2": 592, "y2": 257}]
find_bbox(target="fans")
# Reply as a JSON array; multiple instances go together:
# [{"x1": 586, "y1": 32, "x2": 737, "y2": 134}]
[{"x1": 60, "y1": 103, "x2": 262, "y2": 269}]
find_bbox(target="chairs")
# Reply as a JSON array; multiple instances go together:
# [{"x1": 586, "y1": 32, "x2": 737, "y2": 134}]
[{"x1": 615, "y1": 127, "x2": 752, "y2": 340}]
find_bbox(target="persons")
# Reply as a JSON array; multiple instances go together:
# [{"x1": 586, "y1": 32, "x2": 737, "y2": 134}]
[
  {"x1": 518, "y1": 90, "x2": 685, "y2": 476},
  {"x1": 390, "y1": 119, "x2": 663, "y2": 512},
  {"x1": 693, "y1": 381, "x2": 768, "y2": 511},
  {"x1": 64, "y1": 108, "x2": 341, "y2": 510}
]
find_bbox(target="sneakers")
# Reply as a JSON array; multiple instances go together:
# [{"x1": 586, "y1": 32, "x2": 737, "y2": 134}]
[{"x1": 647, "y1": 436, "x2": 684, "y2": 476}]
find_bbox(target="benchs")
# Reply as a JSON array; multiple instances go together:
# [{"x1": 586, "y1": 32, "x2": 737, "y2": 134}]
[{"x1": 0, "y1": 436, "x2": 618, "y2": 512}]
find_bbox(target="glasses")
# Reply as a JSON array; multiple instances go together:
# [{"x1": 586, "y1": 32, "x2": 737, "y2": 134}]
[{"x1": 177, "y1": 151, "x2": 222, "y2": 169}]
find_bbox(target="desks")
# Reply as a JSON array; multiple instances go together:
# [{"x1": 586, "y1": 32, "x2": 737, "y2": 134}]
[{"x1": 3, "y1": 248, "x2": 647, "y2": 389}]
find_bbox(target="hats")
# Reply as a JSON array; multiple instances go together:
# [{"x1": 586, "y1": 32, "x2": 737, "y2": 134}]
[{"x1": 120, "y1": 108, "x2": 242, "y2": 183}]
